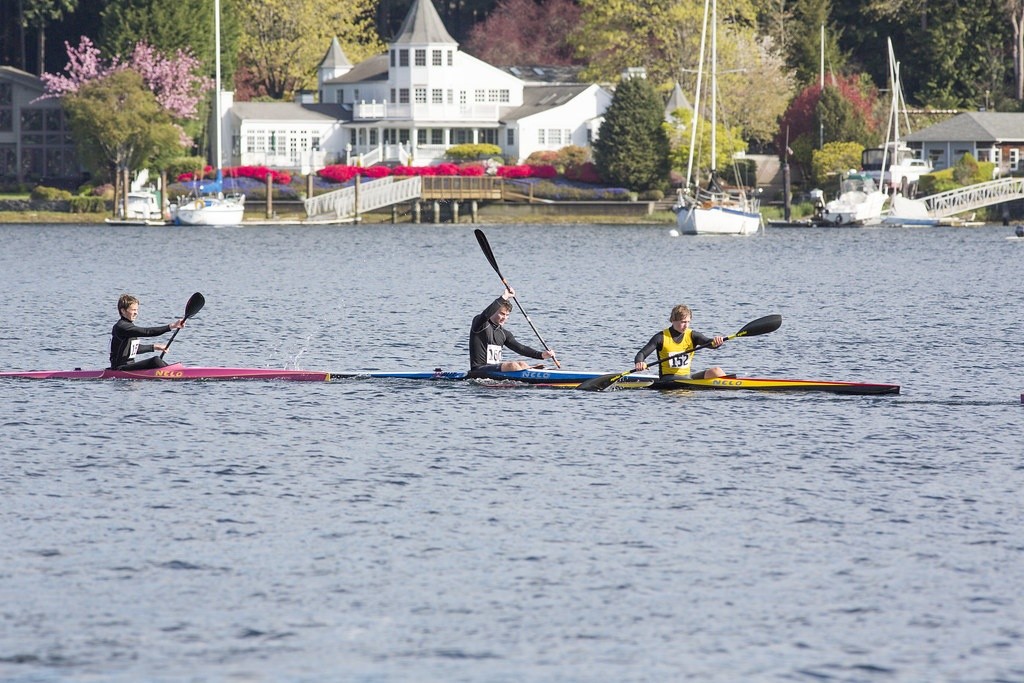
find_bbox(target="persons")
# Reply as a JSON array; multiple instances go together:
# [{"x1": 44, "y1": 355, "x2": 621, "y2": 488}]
[
  {"x1": 634, "y1": 305, "x2": 726, "y2": 378},
  {"x1": 468, "y1": 287, "x2": 556, "y2": 371},
  {"x1": 109, "y1": 293, "x2": 186, "y2": 371}
]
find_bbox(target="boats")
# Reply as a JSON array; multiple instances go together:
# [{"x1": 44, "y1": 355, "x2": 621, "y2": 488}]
[
  {"x1": 881, "y1": 196, "x2": 985, "y2": 227},
  {"x1": 0, "y1": 358, "x2": 335, "y2": 382},
  {"x1": 108, "y1": 186, "x2": 165, "y2": 225},
  {"x1": 327, "y1": 360, "x2": 901, "y2": 396},
  {"x1": 809, "y1": 169, "x2": 889, "y2": 227}
]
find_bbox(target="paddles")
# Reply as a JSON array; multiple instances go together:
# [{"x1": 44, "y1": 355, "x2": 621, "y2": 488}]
[
  {"x1": 576, "y1": 314, "x2": 784, "y2": 392},
  {"x1": 472, "y1": 227, "x2": 564, "y2": 369},
  {"x1": 159, "y1": 291, "x2": 206, "y2": 359}
]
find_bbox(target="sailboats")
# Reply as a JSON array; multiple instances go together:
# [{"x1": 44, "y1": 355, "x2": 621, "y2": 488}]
[
  {"x1": 671, "y1": 0, "x2": 764, "y2": 236},
  {"x1": 166, "y1": 0, "x2": 247, "y2": 227},
  {"x1": 860, "y1": 35, "x2": 932, "y2": 194}
]
